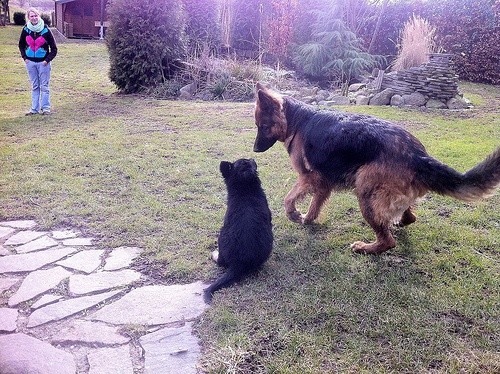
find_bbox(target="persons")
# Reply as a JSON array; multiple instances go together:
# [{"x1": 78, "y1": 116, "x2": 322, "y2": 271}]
[{"x1": 17, "y1": 6, "x2": 57, "y2": 115}]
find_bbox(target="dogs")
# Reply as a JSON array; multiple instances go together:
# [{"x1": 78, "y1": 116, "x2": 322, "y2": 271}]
[
  {"x1": 253, "y1": 81, "x2": 500, "y2": 256},
  {"x1": 203, "y1": 158, "x2": 273, "y2": 306}
]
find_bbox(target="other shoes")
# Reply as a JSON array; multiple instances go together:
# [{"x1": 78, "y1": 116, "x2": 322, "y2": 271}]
[
  {"x1": 26, "y1": 112, "x2": 38, "y2": 116},
  {"x1": 43, "y1": 112, "x2": 50, "y2": 115}
]
